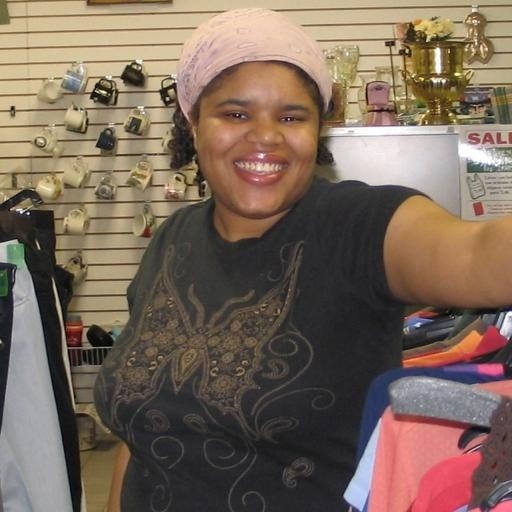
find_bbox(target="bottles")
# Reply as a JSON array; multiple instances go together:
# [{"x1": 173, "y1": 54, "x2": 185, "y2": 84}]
[
  {"x1": 321, "y1": 54, "x2": 345, "y2": 124},
  {"x1": 64, "y1": 315, "x2": 85, "y2": 367}
]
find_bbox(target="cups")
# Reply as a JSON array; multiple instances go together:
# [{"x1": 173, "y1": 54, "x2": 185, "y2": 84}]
[
  {"x1": 27, "y1": 62, "x2": 152, "y2": 156},
  {"x1": 160, "y1": 129, "x2": 175, "y2": 154},
  {"x1": 130, "y1": 204, "x2": 157, "y2": 238},
  {"x1": 126, "y1": 160, "x2": 155, "y2": 192},
  {"x1": 36, "y1": 175, "x2": 62, "y2": 200},
  {"x1": 62, "y1": 208, "x2": 91, "y2": 234},
  {"x1": 159, "y1": 77, "x2": 177, "y2": 106},
  {"x1": 61, "y1": 256, "x2": 89, "y2": 285},
  {"x1": 93, "y1": 174, "x2": 118, "y2": 200},
  {"x1": 62, "y1": 156, "x2": 90, "y2": 189},
  {"x1": 163, "y1": 173, "x2": 188, "y2": 201}
]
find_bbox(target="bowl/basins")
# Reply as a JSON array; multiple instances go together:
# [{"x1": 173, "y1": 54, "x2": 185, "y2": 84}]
[{"x1": 82, "y1": 323, "x2": 125, "y2": 365}]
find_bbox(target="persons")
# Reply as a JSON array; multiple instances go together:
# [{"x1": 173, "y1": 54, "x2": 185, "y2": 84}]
[{"x1": 93, "y1": 8, "x2": 512, "y2": 512}]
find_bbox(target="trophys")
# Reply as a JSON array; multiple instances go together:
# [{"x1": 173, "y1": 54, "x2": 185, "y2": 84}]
[{"x1": 401, "y1": 39, "x2": 475, "y2": 126}]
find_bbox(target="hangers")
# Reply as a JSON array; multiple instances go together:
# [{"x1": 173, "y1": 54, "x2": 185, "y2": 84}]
[{"x1": 387, "y1": 304, "x2": 512, "y2": 512}]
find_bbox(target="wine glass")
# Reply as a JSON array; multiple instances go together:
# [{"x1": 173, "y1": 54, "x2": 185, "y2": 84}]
[{"x1": 332, "y1": 44, "x2": 360, "y2": 124}]
